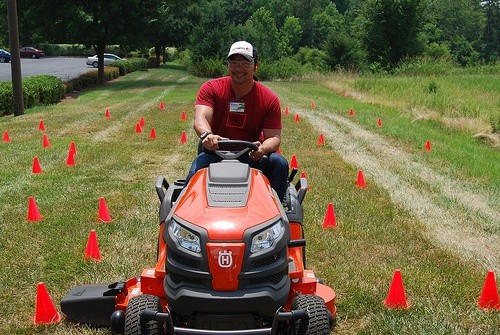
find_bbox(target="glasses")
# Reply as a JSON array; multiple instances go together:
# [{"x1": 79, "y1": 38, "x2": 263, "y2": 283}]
[{"x1": 228, "y1": 59, "x2": 254, "y2": 68}]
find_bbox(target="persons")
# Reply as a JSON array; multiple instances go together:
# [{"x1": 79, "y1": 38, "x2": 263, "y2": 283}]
[{"x1": 183, "y1": 41, "x2": 288, "y2": 203}]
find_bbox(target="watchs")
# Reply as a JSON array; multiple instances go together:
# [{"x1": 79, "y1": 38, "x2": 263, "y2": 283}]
[{"x1": 200, "y1": 131, "x2": 213, "y2": 138}]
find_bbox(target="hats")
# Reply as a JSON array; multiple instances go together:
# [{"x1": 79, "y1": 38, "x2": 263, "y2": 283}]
[{"x1": 227, "y1": 41, "x2": 257, "y2": 62}]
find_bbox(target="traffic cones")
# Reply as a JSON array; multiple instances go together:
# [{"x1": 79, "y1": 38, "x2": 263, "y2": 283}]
[
  {"x1": 84, "y1": 229, "x2": 102, "y2": 262},
  {"x1": 384, "y1": 269, "x2": 408, "y2": 309},
  {"x1": 322, "y1": 203, "x2": 336, "y2": 228},
  {"x1": 34, "y1": 281, "x2": 60, "y2": 326},
  {"x1": 425, "y1": 141, "x2": 431, "y2": 151},
  {"x1": 27, "y1": 197, "x2": 42, "y2": 222},
  {"x1": 66, "y1": 141, "x2": 77, "y2": 165},
  {"x1": 3, "y1": 131, "x2": 10, "y2": 142},
  {"x1": 98, "y1": 197, "x2": 112, "y2": 222},
  {"x1": 39, "y1": 121, "x2": 51, "y2": 147},
  {"x1": 32, "y1": 157, "x2": 42, "y2": 173},
  {"x1": 105, "y1": 102, "x2": 187, "y2": 143},
  {"x1": 478, "y1": 271, "x2": 500, "y2": 312},
  {"x1": 276, "y1": 147, "x2": 306, "y2": 178},
  {"x1": 284, "y1": 103, "x2": 382, "y2": 145},
  {"x1": 357, "y1": 171, "x2": 366, "y2": 186}
]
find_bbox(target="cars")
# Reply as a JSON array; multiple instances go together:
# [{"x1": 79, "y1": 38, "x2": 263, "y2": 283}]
[
  {"x1": 0, "y1": 49, "x2": 11, "y2": 63},
  {"x1": 20, "y1": 47, "x2": 45, "y2": 59},
  {"x1": 87, "y1": 53, "x2": 122, "y2": 68}
]
